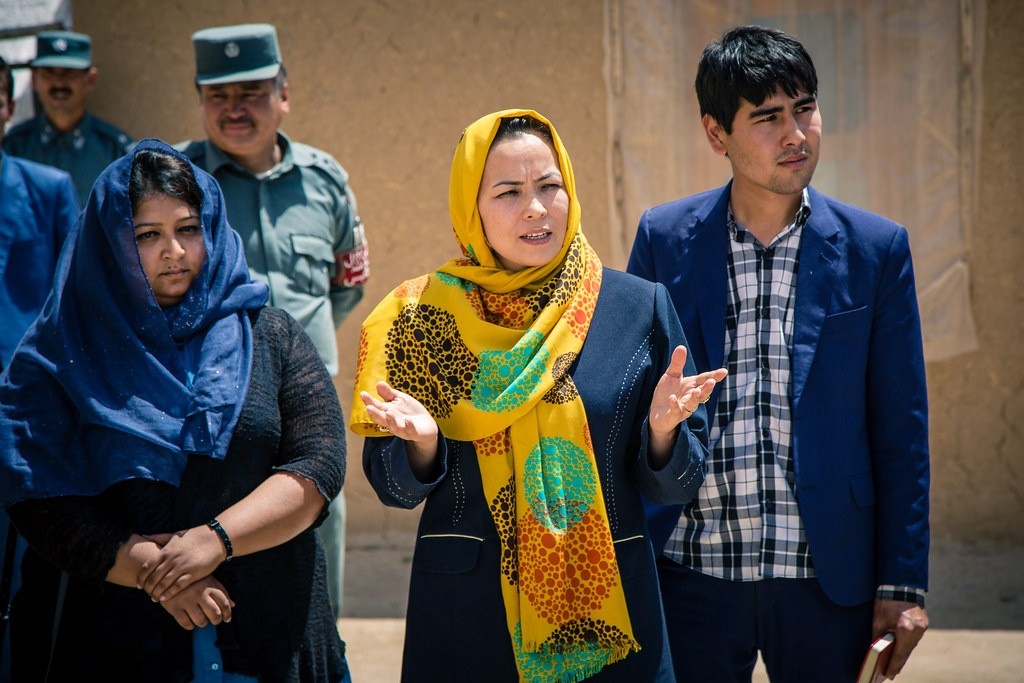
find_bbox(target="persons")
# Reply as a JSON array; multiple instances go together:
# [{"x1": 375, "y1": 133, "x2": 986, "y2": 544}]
[
  {"x1": 350, "y1": 108, "x2": 729, "y2": 683},
  {"x1": 0, "y1": 137, "x2": 352, "y2": 683},
  {"x1": 0, "y1": 56, "x2": 81, "y2": 383},
  {"x1": 3, "y1": 28, "x2": 133, "y2": 209},
  {"x1": 167, "y1": 23, "x2": 370, "y2": 623},
  {"x1": 624, "y1": 26, "x2": 929, "y2": 683}
]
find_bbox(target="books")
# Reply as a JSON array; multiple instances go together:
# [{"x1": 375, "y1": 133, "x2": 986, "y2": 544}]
[{"x1": 858, "y1": 632, "x2": 896, "y2": 683}]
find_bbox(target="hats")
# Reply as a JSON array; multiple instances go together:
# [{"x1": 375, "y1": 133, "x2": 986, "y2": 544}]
[
  {"x1": 31, "y1": 31, "x2": 93, "y2": 70},
  {"x1": 192, "y1": 23, "x2": 281, "y2": 85}
]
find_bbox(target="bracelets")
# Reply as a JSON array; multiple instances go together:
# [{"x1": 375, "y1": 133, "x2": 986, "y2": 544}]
[{"x1": 208, "y1": 518, "x2": 233, "y2": 561}]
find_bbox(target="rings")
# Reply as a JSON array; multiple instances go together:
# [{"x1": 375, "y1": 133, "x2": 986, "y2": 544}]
[
  {"x1": 377, "y1": 424, "x2": 388, "y2": 432},
  {"x1": 698, "y1": 396, "x2": 711, "y2": 404},
  {"x1": 683, "y1": 401, "x2": 699, "y2": 414}
]
